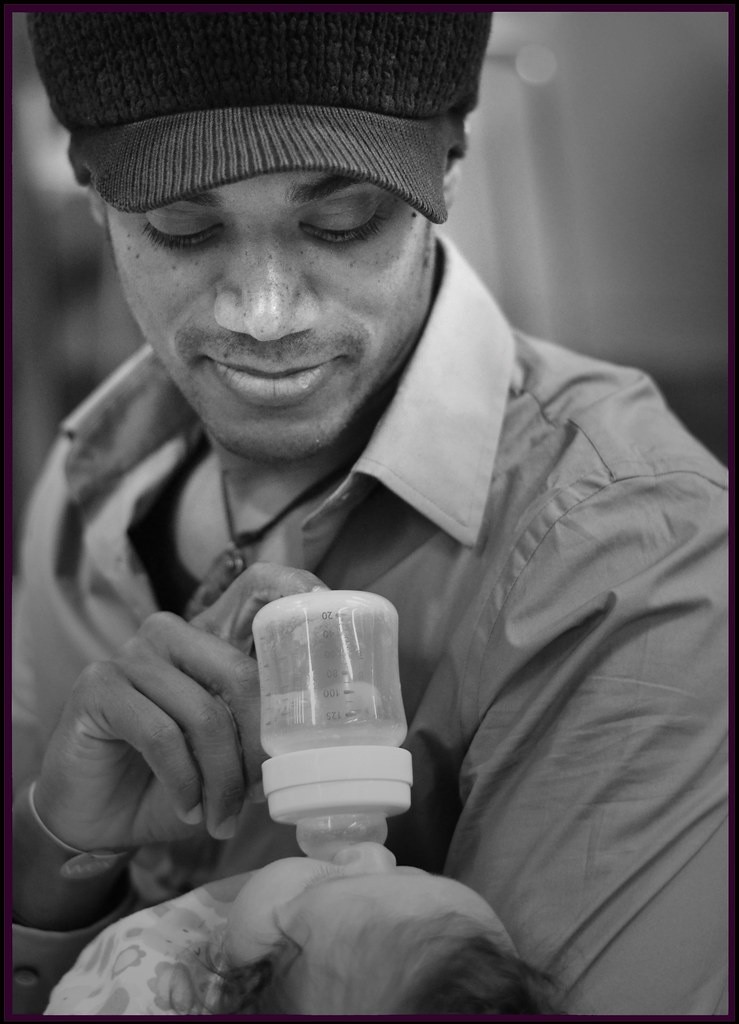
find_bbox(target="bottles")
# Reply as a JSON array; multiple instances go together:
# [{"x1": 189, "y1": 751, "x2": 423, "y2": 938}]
[{"x1": 252, "y1": 590, "x2": 414, "y2": 861}]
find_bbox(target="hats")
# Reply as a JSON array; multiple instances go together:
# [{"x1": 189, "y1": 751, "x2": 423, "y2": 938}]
[{"x1": 26, "y1": 12, "x2": 494, "y2": 224}]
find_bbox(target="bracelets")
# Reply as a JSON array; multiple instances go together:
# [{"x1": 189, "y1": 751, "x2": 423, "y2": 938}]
[{"x1": 29, "y1": 780, "x2": 129, "y2": 881}]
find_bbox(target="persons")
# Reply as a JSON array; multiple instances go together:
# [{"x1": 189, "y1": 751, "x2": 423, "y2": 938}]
[
  {"x1": 11, "y1": 5, "x2": 730, "y2": 1018},
  {"x1": 204, "y1": 841, "x2": 563, "y2": 1018}
]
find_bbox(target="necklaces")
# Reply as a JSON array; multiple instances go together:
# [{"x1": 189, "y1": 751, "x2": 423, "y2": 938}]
[{"x1": 213, "y1": 449, "x2": 362, "y2": 590}]
